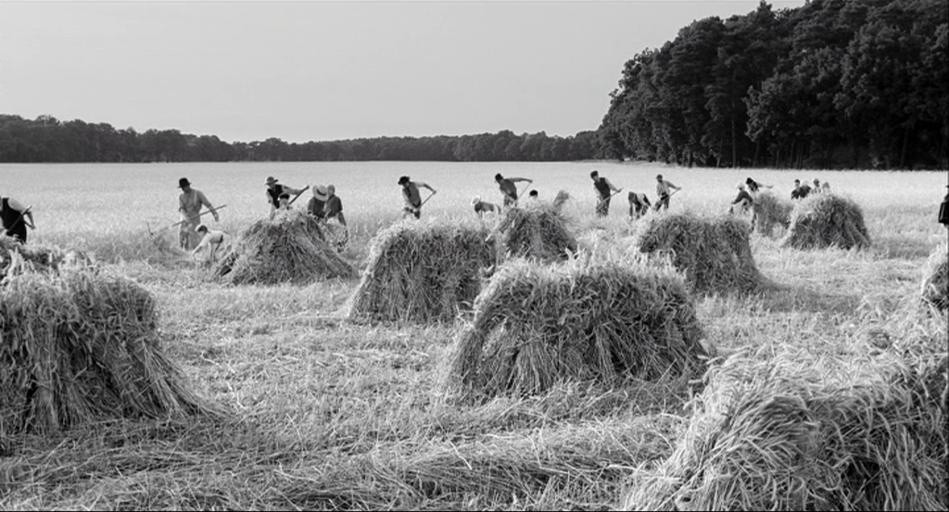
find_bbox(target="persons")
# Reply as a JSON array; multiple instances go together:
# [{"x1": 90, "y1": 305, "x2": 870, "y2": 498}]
[
  {"x1": 177, "y1": 178, "x2": 219, "y2": 247},
  {"x1": 495, "y1": 173, "x2": 533, "y2": 206},
  {"x1": 590, "y1": 170, "x2": 619, "y2": 217},
  {"x1": 728, "y1": 177, "x2": 830, "y2": 214},
  {"x1": 628, "y1": 192, "x2": 651, "y2": 220},
  {"x1": 277, "y1": 193, "x2": 293, "y2": 212},
  {"x1": 191, "y1": 225, "x2": 231, "y2": 259},
  {"x1": 265, "y1": 176, "x2": 302, "y2": 215},
  {"x1": 0, "y1": 196, "x2": 35, "y2": 242},
  {"x1": 398, "y1": 176, "x2": 435, "y2": 219},
  {"x1": 471, "y1": 195, "x2": 501, "y2": 214},
  {"x1": 654, "y1": 174, "x2": 678, "y2": 211},
  {"x1": 326, "y1": 185, "x2": 347, "y2": 225},
  {"x1": 530, "y1": 190, "x2": 538, "y2": 198},
  {"x1": 307, "y1": 185, "x2": 330, "y2": 219}
]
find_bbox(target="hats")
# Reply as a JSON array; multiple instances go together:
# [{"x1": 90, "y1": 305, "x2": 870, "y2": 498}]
[
  {"x1": 177, "y1": 177, "x2": 192, "y2": 188},
  {"x1": 529, "y1": 190, "x2": 538, "y2": 196},
  {"x1": 312, "y1": 184, "x2": 329, "y2": 202},
  {"x1": 264, "y1": 176, "x2": 278, "y2": 185},
  {"x1": 494, "y1": 173, "x2": 503, "y2": 183},
  {"x1": 470, "y1": 195, "x2": 481, "y2": 206},
  {"x1": 398, "y1": 176, "x2": 410, "y2": 184}
]
